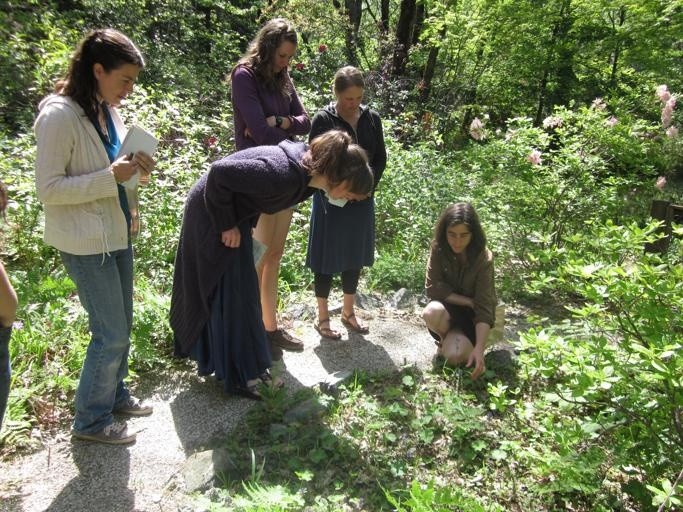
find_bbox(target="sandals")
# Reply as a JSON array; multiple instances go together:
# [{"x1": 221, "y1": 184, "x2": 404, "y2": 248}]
[
  {"x1": 341, "y1": 308, "x2": 369, "y2": 332},
  {"x1": 314, "y1": 315, "x2": 341, "y2": 339}
]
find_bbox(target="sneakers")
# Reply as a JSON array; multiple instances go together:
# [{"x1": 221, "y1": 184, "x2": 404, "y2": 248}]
[
  {"x1": 265, "y1": 328, "x2": 303, "y2": 350},
  {"x1": 118, "y1": 395, "x2": 153, "y2": 416},
  {"x1": 72, "y1": 419, "x2": 137, "y2": 444}
]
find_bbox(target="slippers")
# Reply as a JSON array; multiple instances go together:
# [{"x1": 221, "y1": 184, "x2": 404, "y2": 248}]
[
  {"x1": 231, "y1": 379, "x2": 263, "y2": 401},
  {"x1": 260, "y1": 368, "x2": 284, "y2": 388}
]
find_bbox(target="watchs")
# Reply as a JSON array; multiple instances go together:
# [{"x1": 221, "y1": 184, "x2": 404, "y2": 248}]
[{"x1": 275, "y1": 115, "x2": 283, "y2": 128}]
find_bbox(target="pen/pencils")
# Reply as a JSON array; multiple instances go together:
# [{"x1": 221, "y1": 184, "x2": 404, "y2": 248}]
[{"x1": 119, "y1": 152, "x2": 134, "y2": 185}]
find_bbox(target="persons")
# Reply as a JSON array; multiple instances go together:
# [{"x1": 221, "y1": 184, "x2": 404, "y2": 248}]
[
  {"x1": 305, "y1": 65, "x2": 387, "y2": 340},
  {"x1": 229, "y1": 18, "x2": 311, "y2": 361},
  {"x1": 421, "y1": 202, "x2": 497, "y2": 382},
  {"x1": 1, "y1": 182, "x2": 19, "y2": 437},
  {"x1": 33, "y1": 29, "x2": 158, "y2": 446},
  {"x1": 169, "y1": 130, "x2": 374, "y2": 400}
]
